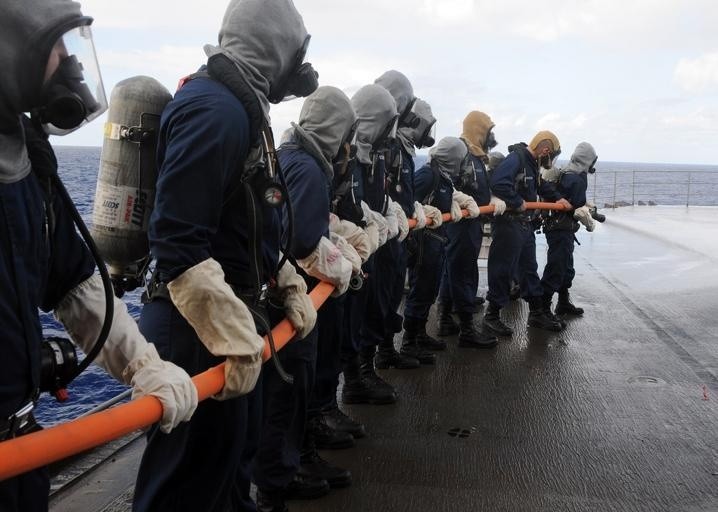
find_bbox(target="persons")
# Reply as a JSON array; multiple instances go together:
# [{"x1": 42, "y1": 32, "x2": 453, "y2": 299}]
[{"x1": 0, "y1": 0, "x2": 600, "y2": 512}]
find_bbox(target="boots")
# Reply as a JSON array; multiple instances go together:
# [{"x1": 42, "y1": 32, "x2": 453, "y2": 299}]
[
  {"x1": 528, "y1": 287, "x2": 584, "y2": 331},
  {"x1": 252, "y1": 448, "x2": 352, "y2": 512},
  {"x1": 307, "y1": 383, "x2": 366, "y2": 449},
  {"x1": 341, "y1": 344, "x2": 398, "y2": 405},
  {"x1": 375, "y1": 322, "x2": 444, "y2": 369},
  {"x1": 438, "y1": 302, "x2": 513, "y2": 347}
]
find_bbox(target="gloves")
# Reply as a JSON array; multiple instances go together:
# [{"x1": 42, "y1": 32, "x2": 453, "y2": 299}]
[
  {"x1": 573, "y1": 206, "x2": 595, "y2": 232},
  {"x1": 53, "y1": 273, "x2": 198, "y2": 434},
  {"x1": 489, "y1": 195, "x2": 506, "y2": 217},
  {"x1": 167, "y1": 257, "x2": 264, "y2": 401},
  {"x1": 276, "y1": 250, "x2": 317, "y2": 339},
  {"x1": 450, "y1": 187, "x2": 480, "y2": 223},
  {"x1": 413, "y1": 201, "x2": 443, "y2": 230},
  {"x1": 296, "y1": 194, "x2": 409, "y2": 297}
]
